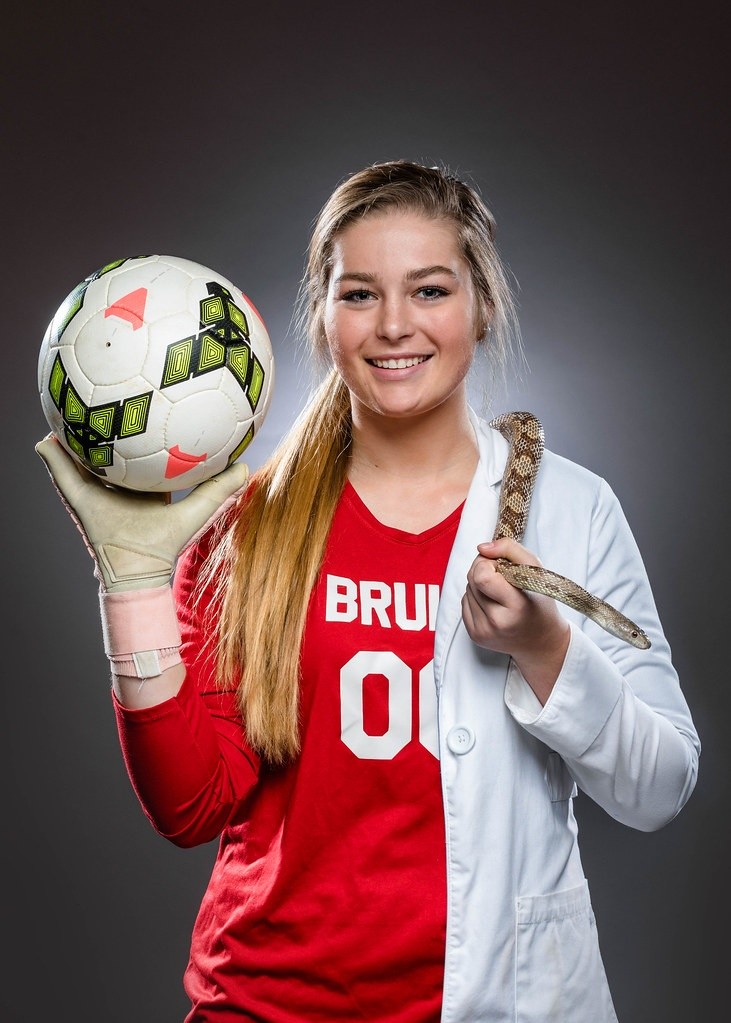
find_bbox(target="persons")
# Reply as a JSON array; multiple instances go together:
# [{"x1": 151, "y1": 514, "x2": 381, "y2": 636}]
[{"x1": 32, "y1": 160, "x2": 700, "y2": 1023}]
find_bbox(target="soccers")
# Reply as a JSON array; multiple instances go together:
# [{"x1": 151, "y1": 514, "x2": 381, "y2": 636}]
[{"x1": 34, "y1": 251, "x2": 277, "y2": 495}]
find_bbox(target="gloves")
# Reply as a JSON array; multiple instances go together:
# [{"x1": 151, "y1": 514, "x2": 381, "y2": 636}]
[{"x1": 35, "y1": 429, "x2": 250, "y2": 682}]
